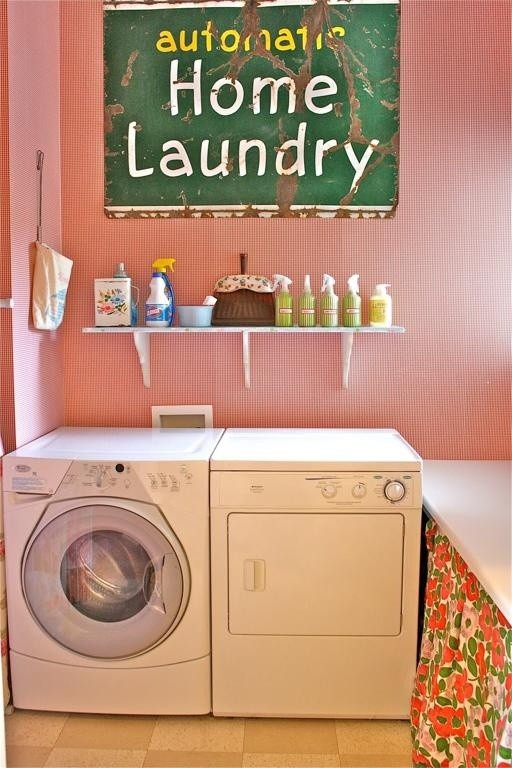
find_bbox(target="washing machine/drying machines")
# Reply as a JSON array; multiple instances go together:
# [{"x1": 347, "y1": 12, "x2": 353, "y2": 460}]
[{"x1": 3, "y1": 426, "x2": 224, "y2": 716}]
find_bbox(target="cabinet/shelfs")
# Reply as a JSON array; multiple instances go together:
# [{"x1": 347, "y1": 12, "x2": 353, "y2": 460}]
[{"x1": 79, "y1": 323, "x2": 405, "y2": 389}]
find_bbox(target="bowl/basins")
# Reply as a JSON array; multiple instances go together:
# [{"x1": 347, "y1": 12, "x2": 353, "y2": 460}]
[{"x1": 177, "y1": 305, "x2": 214, "y2": 326}]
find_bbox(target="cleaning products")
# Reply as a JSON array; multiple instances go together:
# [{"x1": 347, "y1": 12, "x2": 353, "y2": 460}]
[
  {"x1": 320, "y1": 274, "x2": 338, "y2": 329},
  {"x1": 274, "y1": 273, "x2": 293, "y2": 327},
  {"x1": 299, "y1": 277, "x2": 317, "y2": 327},
  {"x1": 115, "y1": 263, "x2": 137, "y2": 327},
  {"x1": 143, "y1": 258, "x2": 175, "y2": 327},
  {"x1": 344, "y1": 274, "x2": 363, "y2": 327}
]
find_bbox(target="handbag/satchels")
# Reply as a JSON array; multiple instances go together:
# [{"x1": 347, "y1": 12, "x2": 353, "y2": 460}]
[{"x1": 31, "y1": 240, "x2": 74, "y2": 332}]
